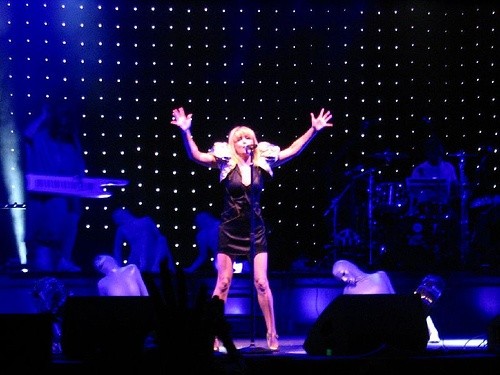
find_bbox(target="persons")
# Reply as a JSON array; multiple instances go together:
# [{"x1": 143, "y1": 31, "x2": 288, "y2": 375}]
[
  {"x1": 112, "y1": 208, "x2": 175, "y2": 272},
  {"x1": 93, "y1": 254, "x2": 149, "y2": 296},
  {"x1": 412, "y1": 140, "x2": 458, "y2": 196},
  {"x1": 332, "y1": 260, "x2": 441, "y2": 344},
  {"x1": 183, "y1": 212, "x2": 250, "y2": 273},
  {"x1": 170, "y1": 106, "x2": 333, "y2": 352}
]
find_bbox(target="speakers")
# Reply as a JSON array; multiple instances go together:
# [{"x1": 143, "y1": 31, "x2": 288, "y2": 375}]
[
  {"x1": 0, "y1": 313, "x2": 51, "y2": 368},
  {"x1": 60, "y1": 296, "x2": 215, "y2": 361},
  {"x1": 302, "y1": 293, "x2": 429, "y2": 355}
]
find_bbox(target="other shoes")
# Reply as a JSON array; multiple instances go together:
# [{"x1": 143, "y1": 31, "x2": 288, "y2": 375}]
[
  {"x1": 267, "y1": 332, "x2": 278, "y2": 350},
  {"x1": 213, "y1": 339, "x2": 219, "y2": 351}
]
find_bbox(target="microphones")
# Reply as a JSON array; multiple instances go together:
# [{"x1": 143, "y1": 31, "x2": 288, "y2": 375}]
[{"x1": 246, "y1": 144, "x2": 257, "y2": 149}]
